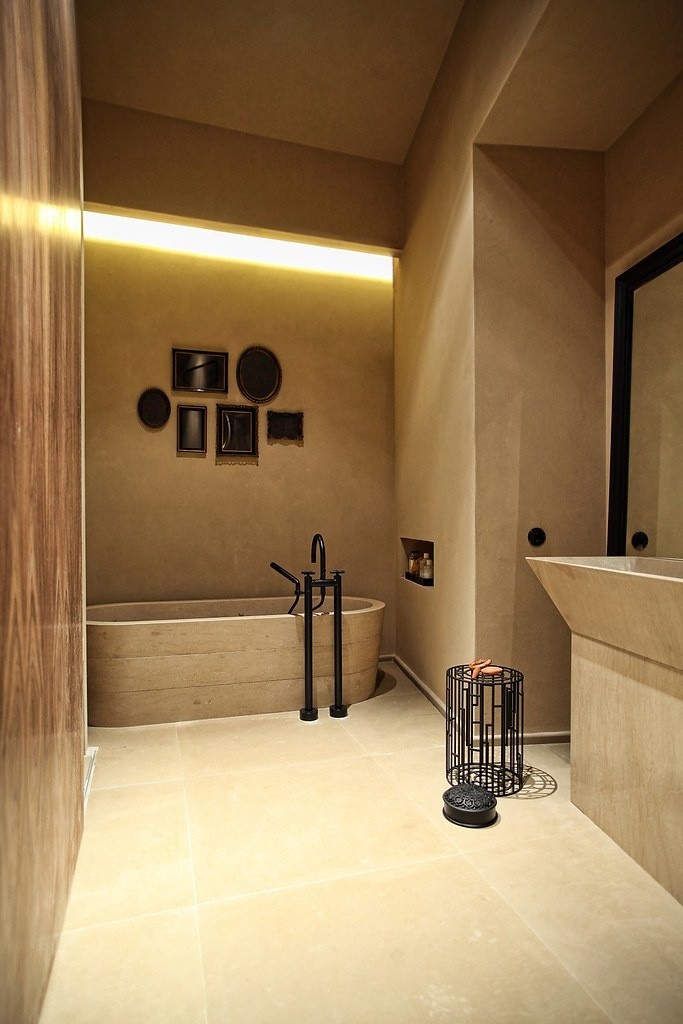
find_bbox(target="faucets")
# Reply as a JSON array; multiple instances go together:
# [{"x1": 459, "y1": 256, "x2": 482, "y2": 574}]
[{"x1": 311, "y1": 534, "x2": 327, "y2": 596}]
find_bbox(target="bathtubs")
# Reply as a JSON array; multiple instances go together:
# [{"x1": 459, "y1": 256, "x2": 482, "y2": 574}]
[{"x1": 86, "y1": 595, "x2": 386, "y2": 728}]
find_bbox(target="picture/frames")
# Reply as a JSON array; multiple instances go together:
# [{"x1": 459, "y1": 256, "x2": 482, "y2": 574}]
[
  {"x1": 267, "y1": 411, "x2": 304, "y2": 441},
  {"x1": 216, "y1": 403, "x2": 259, "y2": 458},
  {"x1": 237, "y1": 346, "x2": 282, "y2": 404},
  {"x1": 172, "y1": 348, "x2": 228, "y2": 393},
  {"x1": 138, "y1": 388, "x2": 171, "y2": 429},
  {"x1": 176, "y1": 405, "x2": 207, "y2": 453}
]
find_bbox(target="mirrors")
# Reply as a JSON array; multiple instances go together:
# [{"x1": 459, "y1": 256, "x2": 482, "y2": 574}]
[{"x1": 607, "y1": 231, "x2": 683, "y2": 559}]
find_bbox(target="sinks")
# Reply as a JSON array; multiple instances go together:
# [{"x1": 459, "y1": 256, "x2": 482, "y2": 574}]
[{"x1": 525, "y1": 557, "x2": 683, "y2": 670}]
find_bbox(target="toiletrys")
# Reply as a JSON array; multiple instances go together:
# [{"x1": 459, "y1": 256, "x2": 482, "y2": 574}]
[{"x1": 405, "y1": 551, "x2": 433, "y2": 579}]
